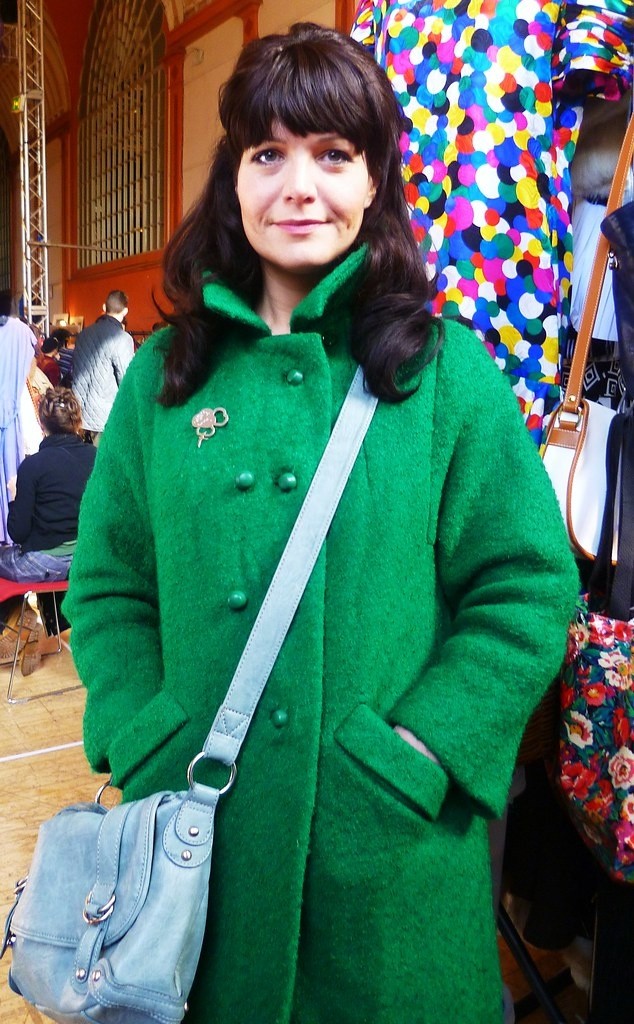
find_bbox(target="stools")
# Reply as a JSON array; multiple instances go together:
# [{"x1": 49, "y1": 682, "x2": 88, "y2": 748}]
[{"x1": 0, "y1": 578, "x2": 85, "y2": 704}]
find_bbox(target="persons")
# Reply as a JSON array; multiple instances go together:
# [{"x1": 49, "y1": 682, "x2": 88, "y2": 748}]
[
  {"x1": 62, "y1": 20, "x2": 582, "y2": 1024},
  {"x1": 0, "y1": 387, "x2": 97, "y2": 582},
  {"x1": 70, "y1": 289, "x2": 135, "y2": 447},
  {"x1": 25, "y1": 322, "x2": 74, "y2": 400}
]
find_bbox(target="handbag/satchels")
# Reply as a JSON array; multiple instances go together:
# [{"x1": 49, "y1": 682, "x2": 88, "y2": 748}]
[
  {"x1": 537, "y1": 397, "x2": 623, "y2": 565},
  {"x1": 550, "y1": 413, "x2": 634, "y2": 884},
  {"x1": 0, "y1": 782, "x2": 220, "y2": 1024}
]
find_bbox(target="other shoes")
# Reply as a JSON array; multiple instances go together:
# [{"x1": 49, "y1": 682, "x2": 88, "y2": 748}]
[
  {"x1": 0, "y1": 604, "x2": 38, "y2": 664},
  {"x1": 17, "y1": 622, "x2": 44, "y2": 677}
]
